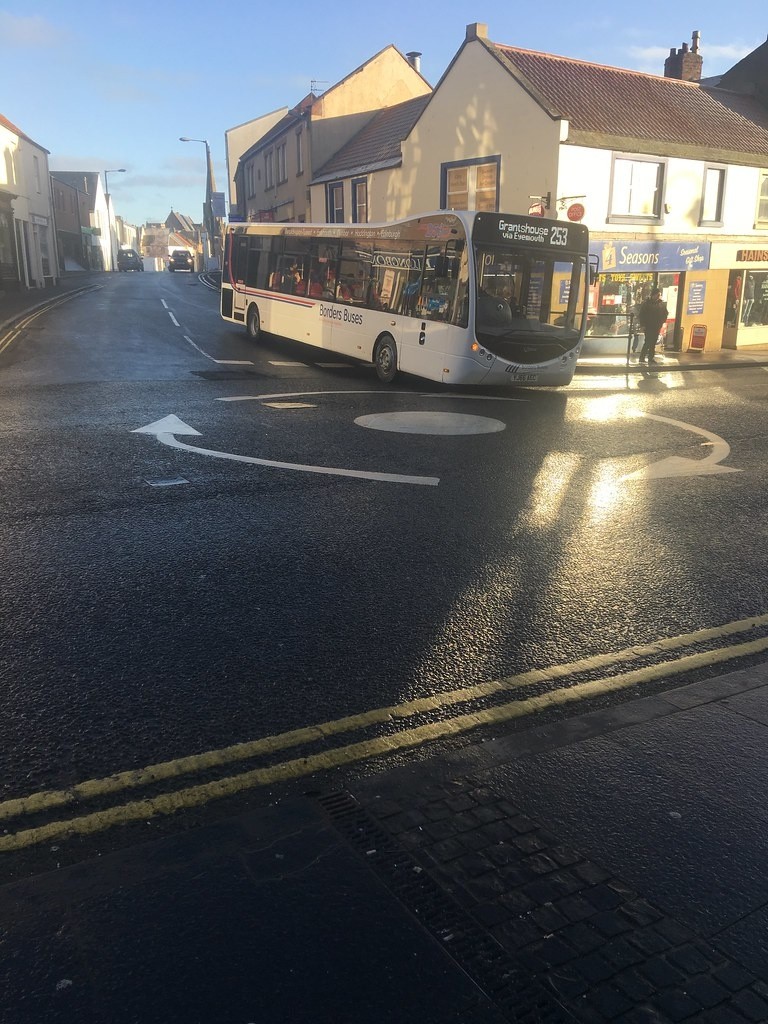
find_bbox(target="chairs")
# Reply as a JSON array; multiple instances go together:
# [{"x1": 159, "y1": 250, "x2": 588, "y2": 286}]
[{"x1": 268, "y1": 272, "x2": 346, "y2": 302}]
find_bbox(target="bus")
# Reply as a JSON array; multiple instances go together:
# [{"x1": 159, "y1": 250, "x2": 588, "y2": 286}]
[{"x1": 220, "y1": 209, "x2": 599, "y2": 387}]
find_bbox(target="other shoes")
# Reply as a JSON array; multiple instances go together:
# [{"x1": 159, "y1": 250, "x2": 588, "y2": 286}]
[
  {"x1": 648, "y1": 359, "x2": 657, "y2": 364},
  {"x1": 639, "y1": 357, "x2": 646, "y2": 362}
]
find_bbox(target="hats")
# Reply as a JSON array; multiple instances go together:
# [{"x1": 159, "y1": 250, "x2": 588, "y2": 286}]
[{"x1": 651, "y1": 288, "x2": 661, "y2": 295}]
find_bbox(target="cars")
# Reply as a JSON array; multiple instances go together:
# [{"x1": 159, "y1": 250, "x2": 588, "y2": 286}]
[
  {"x1": 168, "y1": 250, "x2": 195, "y2": 273},
  {"x1": 117, "y1": 249, "x2": 144, "y2": 272}
]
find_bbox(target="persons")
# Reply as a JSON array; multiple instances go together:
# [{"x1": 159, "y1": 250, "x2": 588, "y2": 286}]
[
  {"x1": 639, "y1": 289, "x2": 668, "y2": 364},
  {"x1": 731, "y1": 274, "x2": 768, "y2": 326}
]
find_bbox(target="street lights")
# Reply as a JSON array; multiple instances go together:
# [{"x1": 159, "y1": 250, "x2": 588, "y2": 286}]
[
  {"x1": 179, "y1": 137, "x2": 215, "y2": 258},
  {"x1": 105, "y1": 169, "x2": 126, "y2": 271}
]
